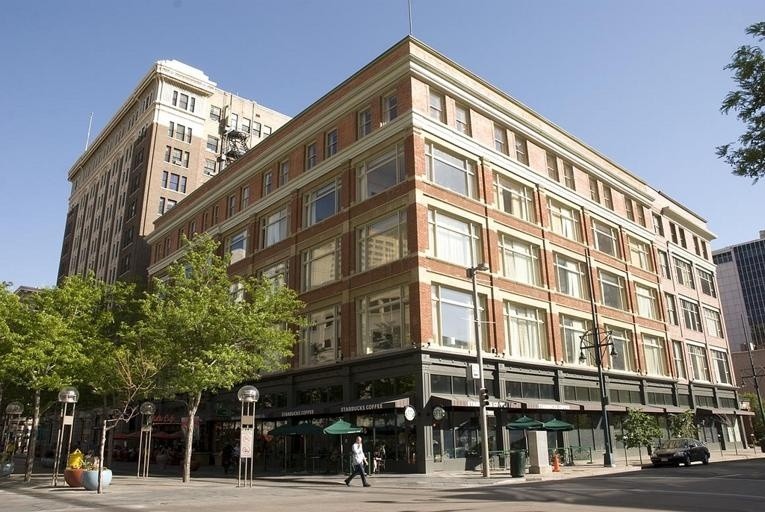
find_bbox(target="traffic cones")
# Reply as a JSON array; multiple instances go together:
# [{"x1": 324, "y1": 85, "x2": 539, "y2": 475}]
[{"x1": 552, "y1": 455, "x2": 561, "y2": 472}]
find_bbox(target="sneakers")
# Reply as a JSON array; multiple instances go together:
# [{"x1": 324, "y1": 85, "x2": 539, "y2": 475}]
[{"x1": 345, "y1": 479, "x2": 372, "y2": 487}]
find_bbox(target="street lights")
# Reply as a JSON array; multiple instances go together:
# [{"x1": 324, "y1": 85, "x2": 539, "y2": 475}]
[
  {"x1": 85, "y1": 112, "x2": 94, "y2": 151},
  {"x1": 579, "y1": 328, "x2": 618, "y2": 467},
  {"x1": 741, "y1": 375, "x2": 765, "y2": 426},
  {"x1": 466, "y1": 263, "x2": 490, "y2": 477}
]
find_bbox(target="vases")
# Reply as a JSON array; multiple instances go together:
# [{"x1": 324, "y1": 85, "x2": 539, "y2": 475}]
[{"x1": 62, "y1": 468, "x2": 113, "y2": 490}]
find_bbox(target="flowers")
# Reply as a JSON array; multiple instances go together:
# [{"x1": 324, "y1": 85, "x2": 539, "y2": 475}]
[{"x1": 64, "y1": 448, "x2": 112, "y2": 469}]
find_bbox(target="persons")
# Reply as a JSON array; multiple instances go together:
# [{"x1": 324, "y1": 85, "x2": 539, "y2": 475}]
[
  {"x1": 217, "y1": 441, "x2": 232, "y2": 475},
  {"x1": 232, "y1": 441, "x2": 239, "y2": 475},
  {"x1": 344, "y1": 435, "x2": 371, "y2": 488},
  {"x1": 179, "y1": 447, "x2": 185, "y2": 476}
]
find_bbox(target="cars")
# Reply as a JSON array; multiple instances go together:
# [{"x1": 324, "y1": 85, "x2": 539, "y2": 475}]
[{"x1": 651, "y1": 437, "x2": 710, "y2": 467}]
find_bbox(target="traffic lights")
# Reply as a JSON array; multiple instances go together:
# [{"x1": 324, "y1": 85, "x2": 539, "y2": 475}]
[{"x1": 480, "y1": 388, "x2": 489, "y2": 407}]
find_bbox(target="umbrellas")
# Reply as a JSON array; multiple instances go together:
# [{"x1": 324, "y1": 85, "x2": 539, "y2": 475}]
[
  {"x1": 296, "y1": 422, "x2": 322, "y2": 470},
  {"x1": 506, "y1": 414, "x2": 544, "y2": 455},
  {"x1": 319, "y1": 418, "x2": 363, "y2": 472},
  {"x1": 539, "y1": 417, "x2": 575, "y2": 449},
  {"x1": 267, "y1": 423, "x2": 303, "y2": 471}
]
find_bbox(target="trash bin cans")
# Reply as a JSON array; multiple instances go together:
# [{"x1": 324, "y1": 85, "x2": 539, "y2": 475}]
[{"x1": 510, "y1": 451, "x2": 525, "y2": 477}]
